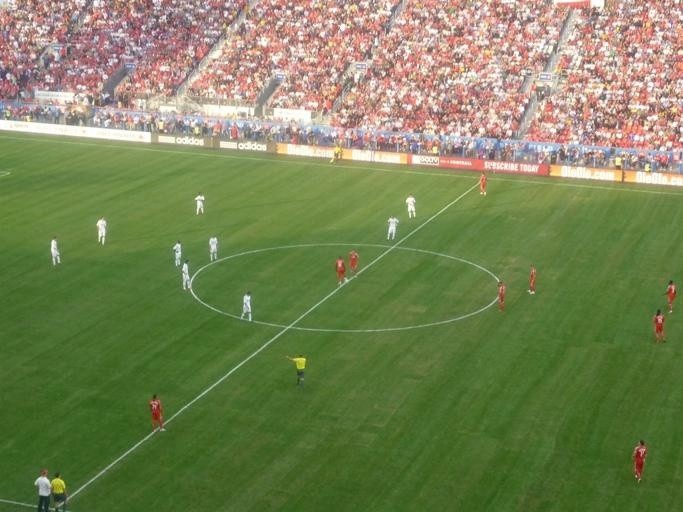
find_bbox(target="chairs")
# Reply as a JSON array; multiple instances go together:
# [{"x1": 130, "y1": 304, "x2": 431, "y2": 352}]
[{"x1": 11, "y1": 0, "x2": 683, "y2": 180}]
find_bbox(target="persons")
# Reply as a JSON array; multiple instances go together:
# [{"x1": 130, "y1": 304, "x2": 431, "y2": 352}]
[
  {"x1": 632, "y1": 440, "x2": 648, "y2": 484},
  {"x1": 147, "y1": 394, "x2": 166, "y2": 432},
  {"x1": 50, "y1": 236, "x2": 61, "y2": 266},
  {"x1": 653, "y1": 309, "x2": 667, "y2": 343},
  {"x1": 349, "y1": 250, "x2": 360, "y2": 273},
  {"x1": 662, "y1": 281, "x2": 677, "y2": 314},
  {"x1": 241, "y1": 292, "x2": 252, "y2": 322},
  {"x1": 405, "y1": 194, "x2": 417, "y2": 219},
  {"x1": 497, "y1": 281, "x2": 506, "y2": 313},
  {"x1": 336, "y1": 256, "x2": 346, "y2": 286},
  {"x1": 172, "y1": 241, "x2": 181, "y2": 267},
  {"x1": 96, "y1": 216, "x2": 106, "y2": 246},
  {"x1": 527, "y1": 263, "x2": 536, "y2": 296},
  {"x1": 209, "y1": 234, "x2": 218, "y2": 261},
  {"x1": 387, "y1": 216, "x2": 399, "y2": 241},
  {"x1": 34, "y1": 467, "x2": 51, "y2": 512},
  {"x1": 285, "y1": 353, "x2": 306, "y2": 388},
  {"x1": 195, "y1": 192, "x2": 205, "y2": 216},
  {"x1": 182, "y1": 261, "x2": 190, "y2": 290},
  {"x1": 0, "y1": 1, "x2": 683, "y2": 172},
  {"x1": 51, "y1": 473, "x2": 68, "y2": 512},
  {"x1": 479, "y1": 172, "x2": 487, "y2": 196}
]
